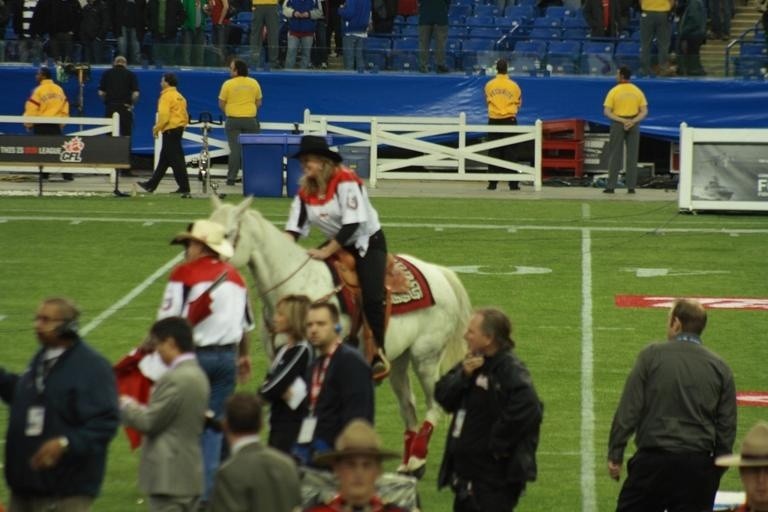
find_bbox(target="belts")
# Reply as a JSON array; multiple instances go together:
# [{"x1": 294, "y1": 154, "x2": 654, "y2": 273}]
[{"x1": 197, "y1": 344, "x2": 236, "y2": 352}]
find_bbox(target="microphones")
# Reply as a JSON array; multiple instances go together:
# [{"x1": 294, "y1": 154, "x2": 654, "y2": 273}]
[{"x1": 292, "y1": 122, "x2": 300, "y2": 135}]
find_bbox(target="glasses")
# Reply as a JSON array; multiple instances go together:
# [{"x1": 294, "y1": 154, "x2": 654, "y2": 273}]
[{"x1": 34, "y1": 315, "x2": 64, "y2": 324}]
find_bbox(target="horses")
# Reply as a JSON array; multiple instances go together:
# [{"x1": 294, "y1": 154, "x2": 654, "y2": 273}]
[{"x1": 204, "y1": 186, "x2": 472, "y2": 479}]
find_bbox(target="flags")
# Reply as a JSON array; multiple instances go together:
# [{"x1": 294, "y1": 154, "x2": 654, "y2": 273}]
[{"x1": 111, "y1": 269, "x2": 227, "y2": 452}]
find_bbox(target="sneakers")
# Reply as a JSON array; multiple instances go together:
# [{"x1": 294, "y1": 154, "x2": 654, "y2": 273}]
[
  {"x1": 170, "y1": 188, "x2": 190, "y2": 193},
  {"x1": 419, "y1": 67, "x2": 431, "y2": 73},
  {"x1": 436, "y1": 65, "x2": 450, "y2": 73},
  {"x1": 136, "y1": 180, "x2": 154, "y2": 192},
  {"x1": 39, "y1": 175, "x2": 49, "y2": 179},
  {"x1": 234, "y1": 178, "x2": 242, "y2": 183},
  {"x1": 329, "y1": 53, "x2": 338, "y2": 57},
  {"x1": 227, "y1": 179, "x2": 234, "y2": 185},
  {"x1": 487, "y1": 185, "x2": 497, "y2": 190},
  {"x1": 321, "y1": 62, "x2": 327, "y2": 69},
  {"x1": 628, "y1": 189, "x2": 636, "y2": 193},
  {"x1": 510, "y1": 186, "x2": 520, "y2": 190},
  {"x1": 370, "y1": 354, "x2": 388, "y2": 380},
  {"x1": 721, "y1": 35, "x2": 730, "y2": 40},
  {"x1": 710, "y1": 35, "x2": 720, "y2": 40},
  {"x1": 308, "y1": 62, "x2": 313, "y2": 69},
  {"x1": 64, "y1": 177, "x2": 73, "y2": 180},
  {"x1": 603, "y1": 189, "x2": 614, "y2": 193}
]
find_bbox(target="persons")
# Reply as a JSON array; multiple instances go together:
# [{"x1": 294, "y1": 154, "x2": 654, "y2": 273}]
[
  {"x1": 716, "y1": 422, "x2": 768, "y2": 512},
  {"x1": 211, "y1": 393, "x2": 300, "y2": 512},
  {"x1": 281, "y1": 0, "x2": 324, "y2": 69},
  {"x1": 484, "y1": 59, "x2": 522, "y2": 192},
  {"x1": 417, "y1": 0, "x2": 452, "y2": 75},
  {"x1": 708, "y1": 0, "x2": 734, "y2": 40},
  {"x1": 152, "y1": 219, "x2": 255, "y2": 497},
  {"x1": 0, "y1": 0, "x2": 179, "y2": 71},
  {"x1": 637, "y1": 0, "x2": 678, "y2": 76},
  {"x1": 678, "y1": 0, "x2": 707, "y2": 76},
  {"x1": 22, "y1": 67, "x2": 74, "y2": 181},
  {"x1": 607, "y1": 299, "x2": 737, "y2": 512},
  {"x1": 288, "y1": 302, "x2": 375, "y2": 466},
  {"x1": 97, "y1": 56, "x2": 140, "y2": 177},
  {"x1": 248, "y1": 0, "x2": 282, "y2": 70},
  {"x1": 218, "y1": 58, "x2": 262, "y2": 186},
  {"x1": 257, "y1": 294, "x2": 315, "y2": 453},
  {"x1": 582, "y1": 0, "x2": 631, "y2": 43},
  {"x1": 337, "y1": 0, "x2": 372, "y2": 71},
  {"x1": 300, "y1": 418, "x2": 411, "y2": 512},
  {"x1": 285, "y1": 135, "x2": 387, "y2": 376},
  {"x1": 181, "y1": 0, "x2": 206, "y2": 66},
  {"x1": 602, "y1": 66, "x2": 648, "y2": 195},
  {"x1": 433, "y1": 308, "x2": 544, "y2": 512},
  {"x1": 346, "y1": 10, "x2": 373, "y2": 70},
  {"x1": 136, "y1": 73, "x2": 191, "y2": 195},
  {"x1": 0, "y1": 298, "x2": 121, "y2": 512},
  {"x1": 208, "y1": 0, "x2": 229, "y2": 68},
  {"x1": 117, "y1": 316, "x2": 210, "y2": 512}
]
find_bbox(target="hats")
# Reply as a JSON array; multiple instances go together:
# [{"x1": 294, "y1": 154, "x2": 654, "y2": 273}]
[
  {"x1": 312, "y1": 422, "x2": 402, "y2": 467},
  {"x1": 715, "y1": 422, "x2": 768, "y2": 466},
  {"x1": 169, "y1": 220, "x2": 234, "y2": 258},
  {"x1": 289, "y1": 135, "x2": 343, "y2": 160}
]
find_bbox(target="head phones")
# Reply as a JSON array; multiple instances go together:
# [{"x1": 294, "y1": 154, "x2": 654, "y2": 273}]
[
  {"x1": 59, "y1": 312, "x2": 80, "y2": 332},
  {"x1": 328, "y1": 320, "x2": 345, "y2": 334}
]
